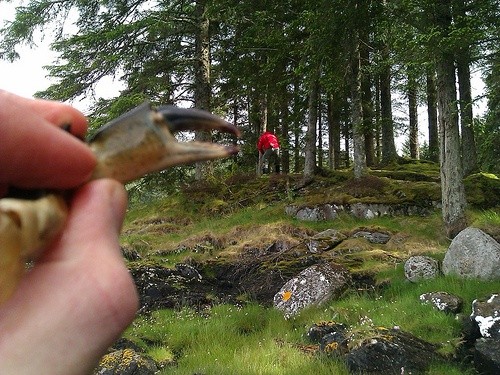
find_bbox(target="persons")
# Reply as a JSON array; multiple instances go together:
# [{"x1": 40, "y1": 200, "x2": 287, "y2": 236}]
[
  {"x1": 0, "y1": 88, "x2": 138, "y2": 375},
  {"x1": 256, "y1": 131, "x2": 284, "y2": 177}
]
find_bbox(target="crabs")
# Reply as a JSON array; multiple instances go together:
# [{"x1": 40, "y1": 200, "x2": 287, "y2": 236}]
[{"x1": 0, "y1": 100, "x2": 244, "y2": 308}]
